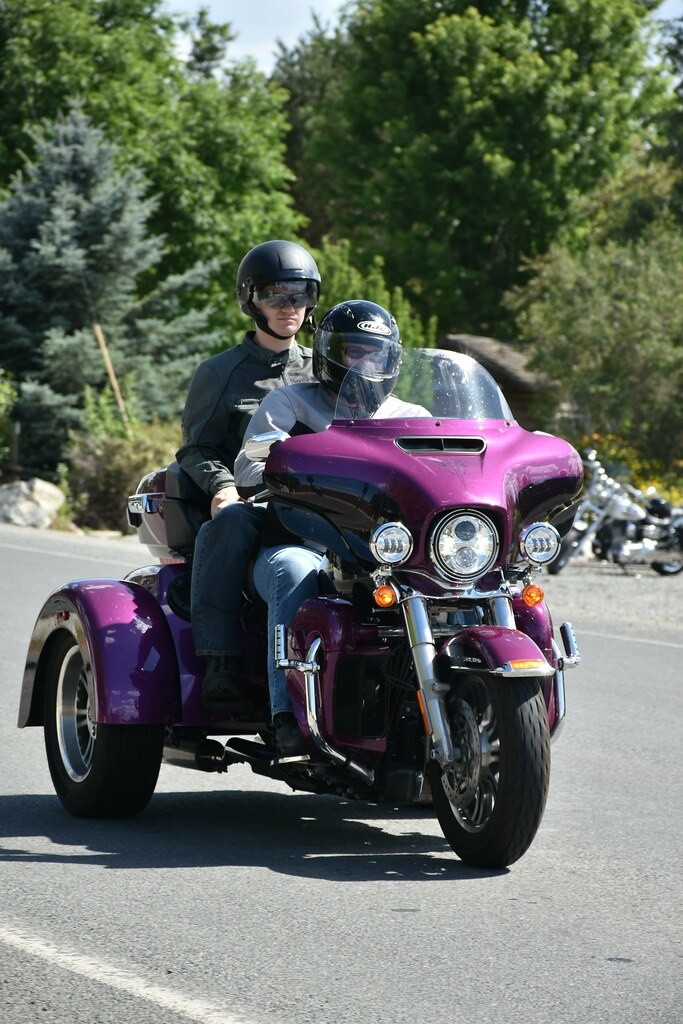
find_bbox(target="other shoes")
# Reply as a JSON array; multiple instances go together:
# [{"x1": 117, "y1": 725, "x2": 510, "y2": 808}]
[{"x1": 275, "y1": 719, "x2": 303, "y2": 756}]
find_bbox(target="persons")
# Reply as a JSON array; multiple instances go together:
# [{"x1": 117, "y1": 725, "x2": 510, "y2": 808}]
[{"x1": 176, "y1": 239, "x2": 434, "y2": 761}]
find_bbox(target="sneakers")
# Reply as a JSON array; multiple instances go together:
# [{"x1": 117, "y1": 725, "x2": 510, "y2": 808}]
[{"x1": 201, "y1": 655, "x2": 238, "y2": 701}]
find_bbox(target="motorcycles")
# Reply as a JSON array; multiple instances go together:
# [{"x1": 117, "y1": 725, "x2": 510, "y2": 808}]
[
  {"x1": 16, "y1": 349, "x2": 586, "y2": 867},
  {"x1": 547, "y1": 448, "x2": 683, "y2": 576}
]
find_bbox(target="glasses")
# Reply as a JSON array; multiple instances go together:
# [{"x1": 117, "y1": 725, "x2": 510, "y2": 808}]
[
  {"x1": 340, "y1": 345, "x2": 390, "y2": 364},
  {"x1": 253, "y1": 291, "x2": 313, "y2": 309}
]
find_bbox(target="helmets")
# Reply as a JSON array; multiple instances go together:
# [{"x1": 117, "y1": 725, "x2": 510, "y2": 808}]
[
  {"x1": 235, "y1": 240, "x2": 323, "y2": 306},
  {"x1": 312, "y1": 299, "x2": 405, "y2": 406}
]
